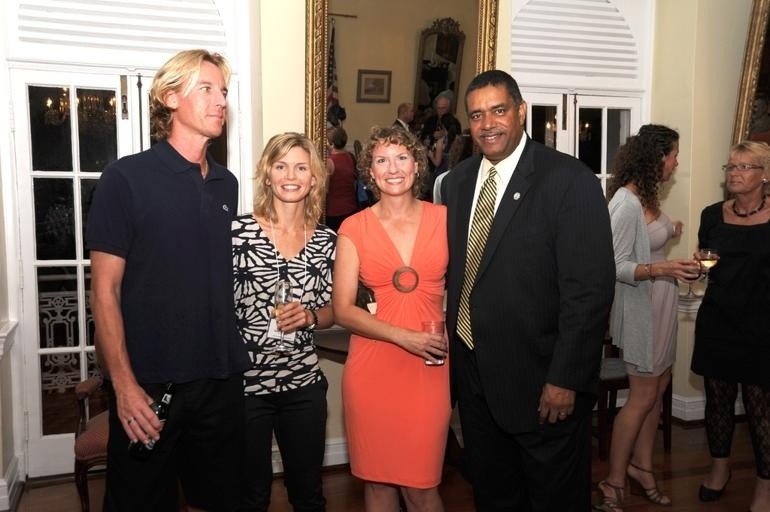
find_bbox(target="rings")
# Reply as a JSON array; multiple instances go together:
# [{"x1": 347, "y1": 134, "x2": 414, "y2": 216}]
[
  {"x1": 559, "y1": 412, "x2": 567, "y2": 416},
  {"x1": 128, "y1": 418, "x2": 135, "y2": 425}
]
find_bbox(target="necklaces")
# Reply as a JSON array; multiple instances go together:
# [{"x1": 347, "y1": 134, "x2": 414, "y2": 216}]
[{"x1": 732, "y1": 195, "x2": 766, "y2": 218}]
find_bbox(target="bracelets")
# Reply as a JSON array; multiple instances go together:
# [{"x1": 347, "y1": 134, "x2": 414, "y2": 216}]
[
  {"x1": 304, "y1": 309, "x2": 318, "y2": 332},
  {"x1": 644, "y1": 264, "x2": 655, "y2": 279}
]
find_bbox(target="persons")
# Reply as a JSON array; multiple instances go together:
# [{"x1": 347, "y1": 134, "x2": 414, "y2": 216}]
[
  {"x1": 696, "y1": 141, "x2": 770, "y2": 512},
  {"x1": 332, "y1": 126, "x2": 450, "y2": 511},
  {"x1": 326, "y1": 97, "x2": 463, "y2": 229},
  {"x1": 443, "y1": 72, "x2": 616, "y2": 511},
  {"x1": 231, "y1": 132, "x2": 338, "y2": 511},
  {"x1": 86, "y1": 48, "x2": 259, "y2": 512},
  {"x1": 609, "y1": 124, "x2": 700, "y2": 511}
]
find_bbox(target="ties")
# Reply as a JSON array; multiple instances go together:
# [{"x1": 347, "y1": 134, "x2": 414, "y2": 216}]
[{"x1": 453, "y1": 167, "x2": 498, "y2": 353}]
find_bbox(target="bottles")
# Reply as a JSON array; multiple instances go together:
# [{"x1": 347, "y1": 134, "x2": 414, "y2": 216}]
[{"x1": 127, "y1": 381, "x2": 177, "y2": 466}]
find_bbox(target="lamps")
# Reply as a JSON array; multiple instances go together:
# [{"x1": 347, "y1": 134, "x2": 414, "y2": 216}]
[{"x1": 45, "y1": 87, "x2": 116, "y2": 125}]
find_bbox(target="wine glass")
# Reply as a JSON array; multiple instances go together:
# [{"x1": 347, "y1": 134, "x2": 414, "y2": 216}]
[
  {"x1": 274, "y1": 281, "x2": 294, "y2": 352},
  {"x1": 678, "y1": 262, "x2": 703, "y2": 300},
  {"x1": 698, "y1": 247, "x2": 718, "y2": 285}
]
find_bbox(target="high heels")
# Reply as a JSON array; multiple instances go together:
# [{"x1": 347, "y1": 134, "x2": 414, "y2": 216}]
[
  {"x1": 698, "y1": 468, "x2": 732, "y2": 500},
  {"x1": 626, "y1": 461, "x2": 673, "y2": 506},
  {"x1": 593, "y1": 479, "x2": 626, "y2": 512}
]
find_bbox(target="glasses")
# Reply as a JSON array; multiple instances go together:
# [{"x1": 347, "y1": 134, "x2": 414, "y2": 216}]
[{"x1": 722, "y1": 163, "x2": 764, "y2": 172}]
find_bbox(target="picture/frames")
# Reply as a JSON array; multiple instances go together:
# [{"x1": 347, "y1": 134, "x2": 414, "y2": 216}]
[
  {"x1": 725, "y1": 0, "x2": 770, "y2": 199},
  {"x1": 355, "y1": 70, "x2": 391, "y2": 103}
]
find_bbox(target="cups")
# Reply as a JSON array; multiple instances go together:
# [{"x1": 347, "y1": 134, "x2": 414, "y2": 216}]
[{"x1": 421, "y1": 319, "x2": 445, "y2": 364}]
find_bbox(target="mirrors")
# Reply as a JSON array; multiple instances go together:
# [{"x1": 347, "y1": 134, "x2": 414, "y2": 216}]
[{"x1": 306, "y1": 1, "x2": 499, "y2": 354}]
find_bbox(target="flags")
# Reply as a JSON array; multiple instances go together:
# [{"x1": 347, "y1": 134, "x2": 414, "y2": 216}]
[{"x1": 327, "y1": 25, "x2": 339, "y2": 105}]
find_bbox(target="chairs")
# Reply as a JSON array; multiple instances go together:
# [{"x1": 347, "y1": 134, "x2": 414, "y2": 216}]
[
  {"x1": 596, "y1": 337, "x2": 672, "y2": 460},
  {"x1": 75, "y1": 376, "x2": 109, "y2": 512}
]
